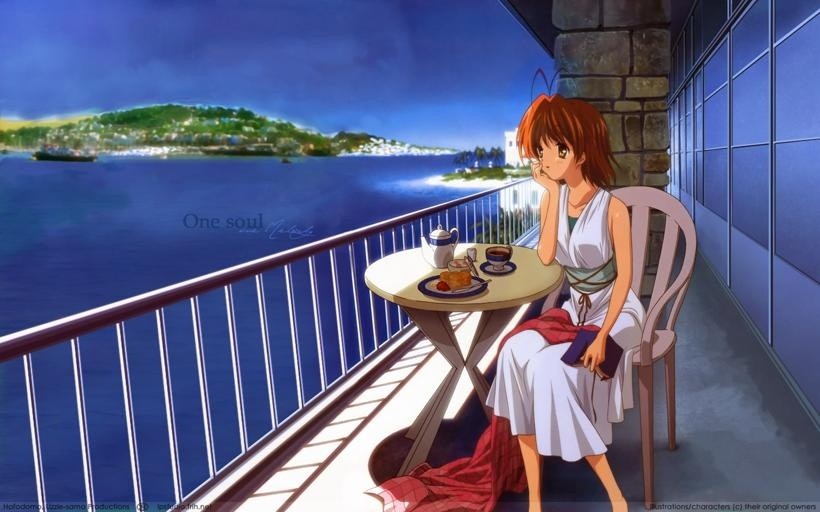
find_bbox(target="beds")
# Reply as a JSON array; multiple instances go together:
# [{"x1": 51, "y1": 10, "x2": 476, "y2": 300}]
[{"x1": 364, "y1": 242, "x2": 564, "y2": 479}]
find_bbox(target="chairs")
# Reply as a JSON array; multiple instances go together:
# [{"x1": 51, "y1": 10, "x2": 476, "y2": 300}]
[{"x1": 541, "y1": 186, "x2": 697, "y2": 511}]
